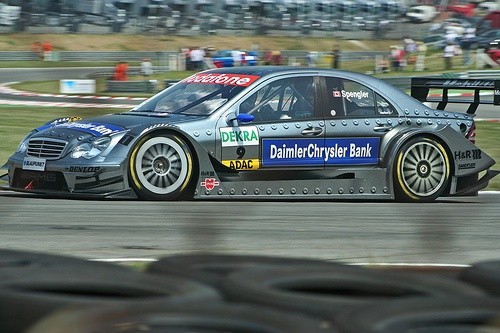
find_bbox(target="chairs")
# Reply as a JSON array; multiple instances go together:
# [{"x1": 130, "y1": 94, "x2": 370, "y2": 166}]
[{"x1": 285, "y1": 96, "x2": 314, "y2": 120}]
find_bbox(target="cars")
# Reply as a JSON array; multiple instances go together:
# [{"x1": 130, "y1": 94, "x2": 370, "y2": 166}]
[
  {"x1": 0, "y1": 66, "x2": 500, "y2": 201},
  {"x1": 211, "y1": 50, "x2": 259, "y2": 66},
  {"x1": 406, "y1": 6, "x2": 500, "y2": 50}
]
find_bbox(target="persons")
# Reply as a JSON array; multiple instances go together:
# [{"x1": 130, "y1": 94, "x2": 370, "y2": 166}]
[
  {"x1": 107, "y1": 44, "x2": 319, "y2": 83},
  {"x1": 378, "y1": 30, "x2": 499, "y2": 73},
  {"x1": 33, "y1": 40, "x2": 54, "y2": 60}
]
